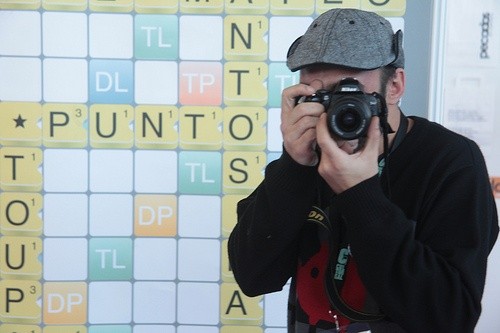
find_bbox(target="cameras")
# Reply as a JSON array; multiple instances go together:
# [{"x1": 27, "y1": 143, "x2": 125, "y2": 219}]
[{"x1": 303, "y1": 78, "x2": 384, "y2": 140}]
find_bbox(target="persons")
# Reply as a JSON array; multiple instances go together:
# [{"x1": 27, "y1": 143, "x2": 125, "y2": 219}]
[{"x1": 227, "y1": 8, "x2": 500, "y2": 333}]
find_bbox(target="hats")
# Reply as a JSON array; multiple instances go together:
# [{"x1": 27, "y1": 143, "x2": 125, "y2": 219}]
[{"x1": 287, "y1": 9, "x2": 405, "y2": 72}]
